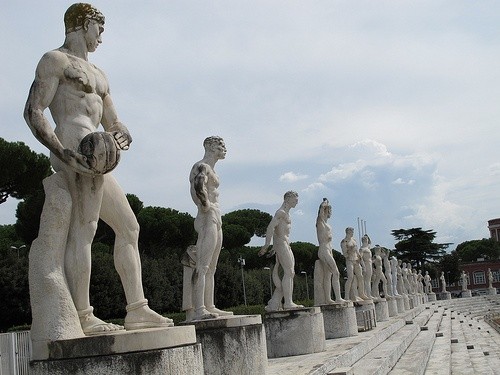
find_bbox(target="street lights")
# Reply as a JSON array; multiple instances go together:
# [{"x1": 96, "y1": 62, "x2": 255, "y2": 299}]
[
  {"x1": 11, "y1": 245, "x2": 26, "y2": 260},
  {"x1": 264, "y1": 267, "x2": 272, "y2": 298},
  {"x1": 301, "y1": 271, "x2": 309, "y2": 300},
  {"x1": 238, "y1": 255, "x2": 247, "y2": 307}
]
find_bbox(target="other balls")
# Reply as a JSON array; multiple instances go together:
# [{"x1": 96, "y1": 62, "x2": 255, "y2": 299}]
[{"x1": 77, "y1": 132, "x2": 121, "y2": 176}]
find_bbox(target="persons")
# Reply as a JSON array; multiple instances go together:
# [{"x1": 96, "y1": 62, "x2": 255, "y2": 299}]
[
  {"x1": 486, "y1": 268, "x2": 494, "y2": 289},
  {"x1": 24, "y1": 2, "x2": 174, "y2": 333},
  {"x1": 461, "y1": 271, "x2": 468, "y2": 290},
  {"x1": 258, "y1": 190, "x2": 304, "y2": 309},
  {"x1": 190, "y1": 135, "x2": 233, "y2": 317},
  {"x1": 340, "y1": 226, "x2": 448, "y2": 303},
  {"x1": 316, "y1": 198, "x2": 351, "y2": 304}
]
